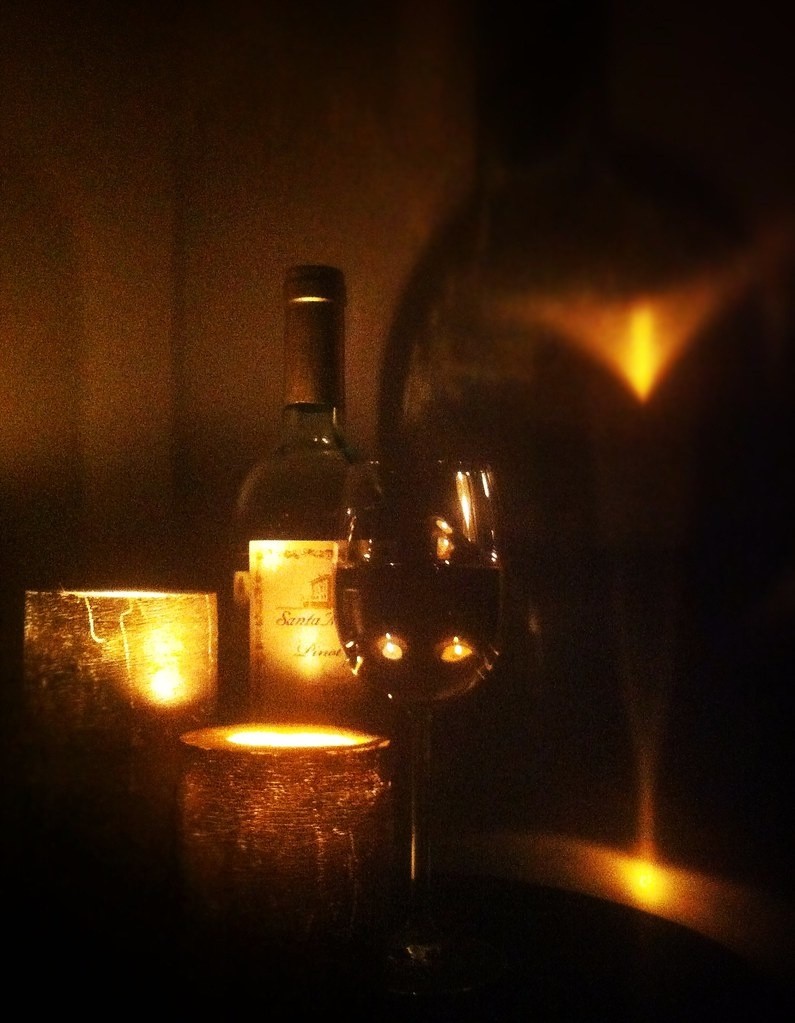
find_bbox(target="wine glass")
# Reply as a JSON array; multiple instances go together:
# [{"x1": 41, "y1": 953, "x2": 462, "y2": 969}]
[{"x1": 331, "y1": 458, "x2": 513, "y2": 962}]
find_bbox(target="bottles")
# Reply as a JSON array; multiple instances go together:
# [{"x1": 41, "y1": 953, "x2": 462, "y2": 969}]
[{"x1": 223, "y1": 263, "x2": 381, "y2": 738}]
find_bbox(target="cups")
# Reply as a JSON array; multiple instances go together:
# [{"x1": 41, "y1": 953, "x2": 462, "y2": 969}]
[{"x1": 20, "y1": 586, "x2": 389, "y2": 936}]
